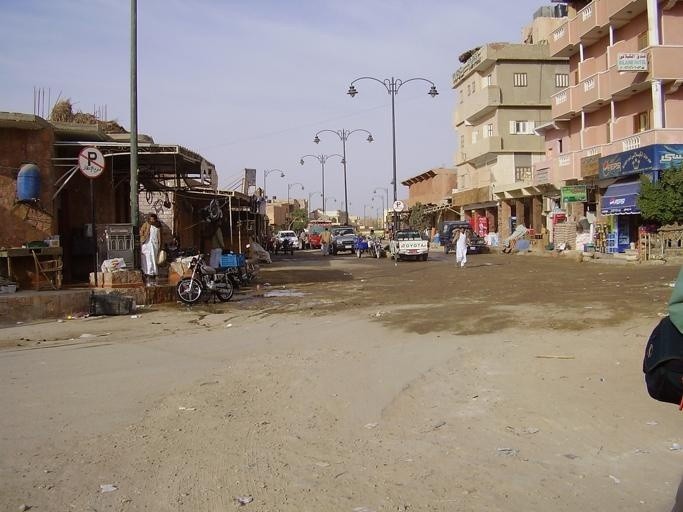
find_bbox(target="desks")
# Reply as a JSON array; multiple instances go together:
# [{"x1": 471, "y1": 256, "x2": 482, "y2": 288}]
[{"x1": 1, "y1": 246, "x2": 63, "y2": 291}]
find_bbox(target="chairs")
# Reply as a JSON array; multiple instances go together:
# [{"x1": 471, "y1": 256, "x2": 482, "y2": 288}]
[{"x1": 31, "y1": 248, "x2": 65, "y2": 291}]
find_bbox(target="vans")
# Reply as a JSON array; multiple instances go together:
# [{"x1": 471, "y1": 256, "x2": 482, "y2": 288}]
[{"x1": 275, "y1": 230, "x2": 299, "y2": 250}]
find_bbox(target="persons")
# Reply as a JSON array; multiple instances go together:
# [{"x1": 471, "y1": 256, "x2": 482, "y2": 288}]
[
  {"x1": 501, "y1": 222, "x2": 528, "y2": 254},
  {"x1": 212, "y1": 218, "x2": 225, "y2": 249},
  {"x1": 368, "y1": 229, "x2": 377, "y2": 249},
  {"x1": 318, "y1": 228, "x2": 331, "y2": 256},
  {"x1": 452, "y1": 227, "x2": 471, "y2": 269},
  {"x1": 139, "y1": 212, "x2": 161, "y2": 287}
]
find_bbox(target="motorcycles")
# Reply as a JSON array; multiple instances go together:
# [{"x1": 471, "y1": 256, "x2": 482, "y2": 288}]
[
  {"x1": 174, "y1": 251, "x2": 234, "y2": 303},
  {"x1": 225, "y1": 243, "x2": 255, "y2": 288}
]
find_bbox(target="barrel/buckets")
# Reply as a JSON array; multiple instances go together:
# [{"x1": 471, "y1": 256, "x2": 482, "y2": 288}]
[{"x1": 16, "y1": 163, "x2": 40, "y2": 199}]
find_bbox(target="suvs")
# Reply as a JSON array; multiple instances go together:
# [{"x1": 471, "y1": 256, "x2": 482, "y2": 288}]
[
  {"x1": 329, "y1": 228, "x2": 359, "y2": 255},
  {"x1": 439, "y1": 220, "x2": 485, "y2": 254}
]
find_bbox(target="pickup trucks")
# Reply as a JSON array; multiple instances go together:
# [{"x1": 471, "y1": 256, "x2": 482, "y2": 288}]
[{"x1": 388, "y1": 229, "x2": 429, "y2": 262}]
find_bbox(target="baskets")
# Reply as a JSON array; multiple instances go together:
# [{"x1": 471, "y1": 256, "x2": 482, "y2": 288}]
[{"x1": 218, "y1": 252, "x2": 246, "y2": 268}]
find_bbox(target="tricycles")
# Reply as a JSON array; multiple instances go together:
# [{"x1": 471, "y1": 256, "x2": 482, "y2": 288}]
[{"x1": 352, "y1": 235, "x2": 386, "y2": 259}]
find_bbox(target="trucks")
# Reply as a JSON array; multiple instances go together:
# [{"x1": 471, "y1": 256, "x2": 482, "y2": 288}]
[{"x1": 303, "y1": 226, "x2": 325, "y2": 249}]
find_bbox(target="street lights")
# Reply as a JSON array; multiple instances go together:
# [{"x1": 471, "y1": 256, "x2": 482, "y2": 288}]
[
  {"x1": 324, "y1": 185, "x2": 389, "y2": 229},
  {"x1": 299, "y1": 154, "x2": 346, "y2": 218},
  {"x1": 313, "y1": 129, "x2": 374, "y2": 224},
  {"x1": 307, "y1": 192, "x2": 322, "y2": 218},
  {"x1": 287, "y1": 182, "x2": 304, "y2": 224},
  {"x1": 263, "y1": 168, "x2": 285, "y2": 214},
  {"x1": 347, "y1": 76, "x2": 439, "y2": 231}
]
